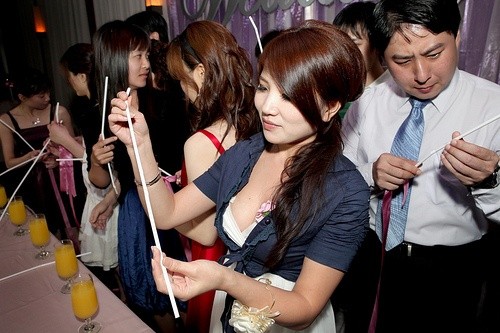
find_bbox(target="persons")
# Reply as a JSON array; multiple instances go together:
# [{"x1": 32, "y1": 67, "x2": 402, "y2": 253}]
[
  {"x1": 108, "y1": 19, "x2": 370, "y2": 332},
  {"x1": 341, "y1": 0, "x2": 500, "y2": 332},
  {"x1": 88, "y1": 19, "x2": 188, "y2": 326},
  {"x1": 47, "y1": 43, "x2": 126, "y2": 302},
  {"x1": 332, "y1": 1, "x2": 386, "y2": 120},
  {"x1": 142, "y1": 40, "x2": 167, "y2": 89},
  {"x1": 156, "y1": 19, "x2": 263, "y2": 333},
  {"x1": 124, "y1": 12, "x2": 169, "y2": 45},
  {"x1": 0, "y1": 74, "x2": 79, "y2": 252}
]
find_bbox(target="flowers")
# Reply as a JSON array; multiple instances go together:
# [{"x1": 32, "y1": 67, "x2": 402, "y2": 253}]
[{"x1": 255, "y1": 200, "x2": 276, "y2": 223}]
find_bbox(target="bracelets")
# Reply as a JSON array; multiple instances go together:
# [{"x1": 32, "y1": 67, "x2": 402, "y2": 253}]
[{"x1": 133, "y1": 171, "x2": 162, "y2": 186}]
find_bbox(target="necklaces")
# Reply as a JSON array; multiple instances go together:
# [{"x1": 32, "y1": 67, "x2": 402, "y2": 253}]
[{"x1": 21, "y1": 108, "x2": 42, "y2": 125}]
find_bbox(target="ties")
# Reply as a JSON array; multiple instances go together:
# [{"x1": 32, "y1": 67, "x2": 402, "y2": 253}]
[{"x1": 375, "y1": 98, "x2": 428, "y2": 252}]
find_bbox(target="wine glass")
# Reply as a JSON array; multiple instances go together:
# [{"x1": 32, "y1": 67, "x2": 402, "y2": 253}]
[
  {"x1": 0, "y1": 187, "x2": 8, "y2": 217},
  {"x1": 8, "y1": 197, "x2": 28, "y2": 237},
  {"x1": 28, "y1": 213, "x2": 54, "y2": 260},
  {"x1": 71, "y1": 272, "x2": 102, "y2": 333},
  {"x1": 53, "y1": 239, "x2": 79, "y2": 294}
]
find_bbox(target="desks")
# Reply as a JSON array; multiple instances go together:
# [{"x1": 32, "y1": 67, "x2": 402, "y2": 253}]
[{"x1": 0, "y1": 198, "x2": 156, "y2": 333}]
